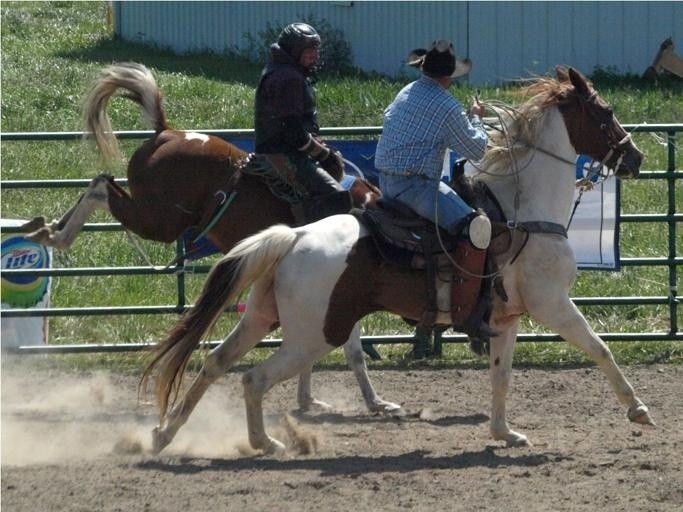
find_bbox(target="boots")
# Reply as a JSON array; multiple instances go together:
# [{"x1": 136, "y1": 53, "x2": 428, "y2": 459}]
[{"x1": 453, "y1": 294, "x2": 500, "y2": 336}]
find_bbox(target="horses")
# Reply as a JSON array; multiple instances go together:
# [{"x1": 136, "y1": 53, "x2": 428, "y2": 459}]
[
  {"x1": 136, "y1": 65, "x2": 658, "y2": 456},
  {"x1": 20, "y1": 61, "x2": 491, "y2": 413}
]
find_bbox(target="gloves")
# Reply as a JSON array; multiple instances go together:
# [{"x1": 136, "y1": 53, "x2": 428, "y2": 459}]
[{"x1": 319, "y1": 149, "x2": 343, "y2": 183}]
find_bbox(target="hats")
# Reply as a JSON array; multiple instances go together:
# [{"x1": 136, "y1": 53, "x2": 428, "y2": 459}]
[{"x1": 407, "y1": 39, "x2": 472, "y2": 79}]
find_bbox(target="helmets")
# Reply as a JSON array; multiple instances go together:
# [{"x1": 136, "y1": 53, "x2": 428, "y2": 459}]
[{"x1": 277, "y1": 23, "x2": 325, "y2": 73}]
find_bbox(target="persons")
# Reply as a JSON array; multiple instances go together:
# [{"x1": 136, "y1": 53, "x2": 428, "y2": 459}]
[
  {"x1": 373, "y1": 37, "x2": 508, "y2": 343},
  {"x1": 251, "y1": 21, "x2": 354, "y2": 222}
]
